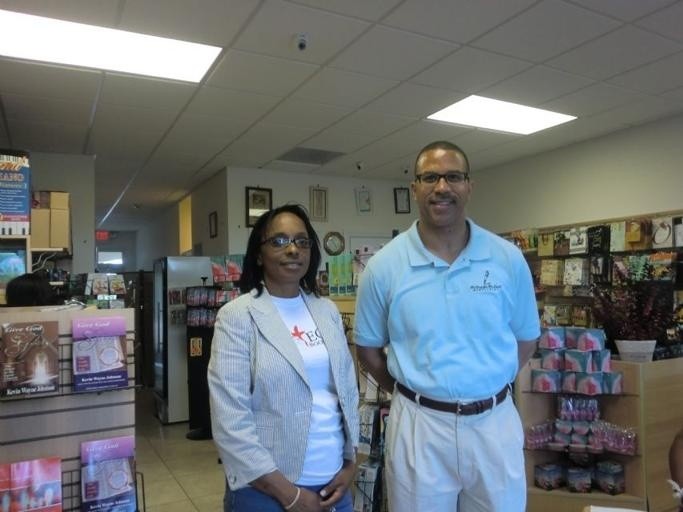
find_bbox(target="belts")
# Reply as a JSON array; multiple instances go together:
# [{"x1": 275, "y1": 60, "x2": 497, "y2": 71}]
[{"x1": 394, "y1": 379, "x2": 509, "y2": 417}]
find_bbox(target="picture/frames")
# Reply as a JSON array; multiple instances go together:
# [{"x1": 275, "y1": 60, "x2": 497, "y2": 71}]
[
  {"x1": 393, "y1": 187, "x2": 410, "y2": 213},
  {"x1": 310, "y1": 186, "x2": 329, "y2": 222},
  {"x1": 209, "y1": 211, "x2": 217, "y2": 238},
  {"x1": 245, "y1": 186, "x2": 272, "y2": 228},
  {"x1": 356, "y1": 187, "x2": 373, "y2": 216}
]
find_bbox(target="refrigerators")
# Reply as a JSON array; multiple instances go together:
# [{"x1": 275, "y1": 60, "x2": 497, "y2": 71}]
[{"x1": 152, "y1": 255, "x2": 214, "y2": 424}]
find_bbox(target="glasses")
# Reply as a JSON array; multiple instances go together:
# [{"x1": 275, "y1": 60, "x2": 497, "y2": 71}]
[
  {"x1": 414, "y1": 170, "x2": 468, "y2": 184},
  {"x1": 256, "y1": 234, "x2": 314, "y2": 251}
]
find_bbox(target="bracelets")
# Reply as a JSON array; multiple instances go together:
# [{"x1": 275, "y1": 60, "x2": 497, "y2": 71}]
[{"x1": 284, "y1": 485, "x2": 300, "y2": 510}]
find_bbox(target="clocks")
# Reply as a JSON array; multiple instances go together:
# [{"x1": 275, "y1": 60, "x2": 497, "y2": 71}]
[{"x1": 323, "y1": 231, "x2": 345, "y2": 256}]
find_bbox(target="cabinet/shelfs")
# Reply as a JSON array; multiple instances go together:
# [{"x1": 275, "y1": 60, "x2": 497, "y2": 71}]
[
  {"x1": 31, "y1": 247, "x2": 71, "y2": 285},
  {"x1": 522, "y1": 391, "x2": 647, "y2": 512}
]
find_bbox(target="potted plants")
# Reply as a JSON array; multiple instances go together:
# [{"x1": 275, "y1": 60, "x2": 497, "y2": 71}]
[{"x1": 589, "y1": 255, "x2": 683, "y2": 354}]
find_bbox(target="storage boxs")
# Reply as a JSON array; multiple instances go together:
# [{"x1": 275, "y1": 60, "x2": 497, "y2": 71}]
[{"x1": 30, "y1": 192, "x2": 73, "y2": 255}]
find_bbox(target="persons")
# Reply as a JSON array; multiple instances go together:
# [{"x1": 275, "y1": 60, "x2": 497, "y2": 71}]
[
  {"x1": 352, "y1": 141, "x2": 540, "y2": 510},
  {"x1": 205, "y1": 204, "x2": 360, "y2": 512}
]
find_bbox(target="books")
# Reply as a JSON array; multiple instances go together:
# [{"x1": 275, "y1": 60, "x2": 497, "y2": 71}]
[
  {"x1": 73, "y1": 315, "x2": 129, "y2": 389},
  {"x1": 0, "y1": 320, "x2": 63, "y2": 403},
  {"x1": 0, "y1": 457, "x2": 62, "y2": 511},
  {"x1": 79, "y1": 435, "x2": 136, "y2": 511}
]
